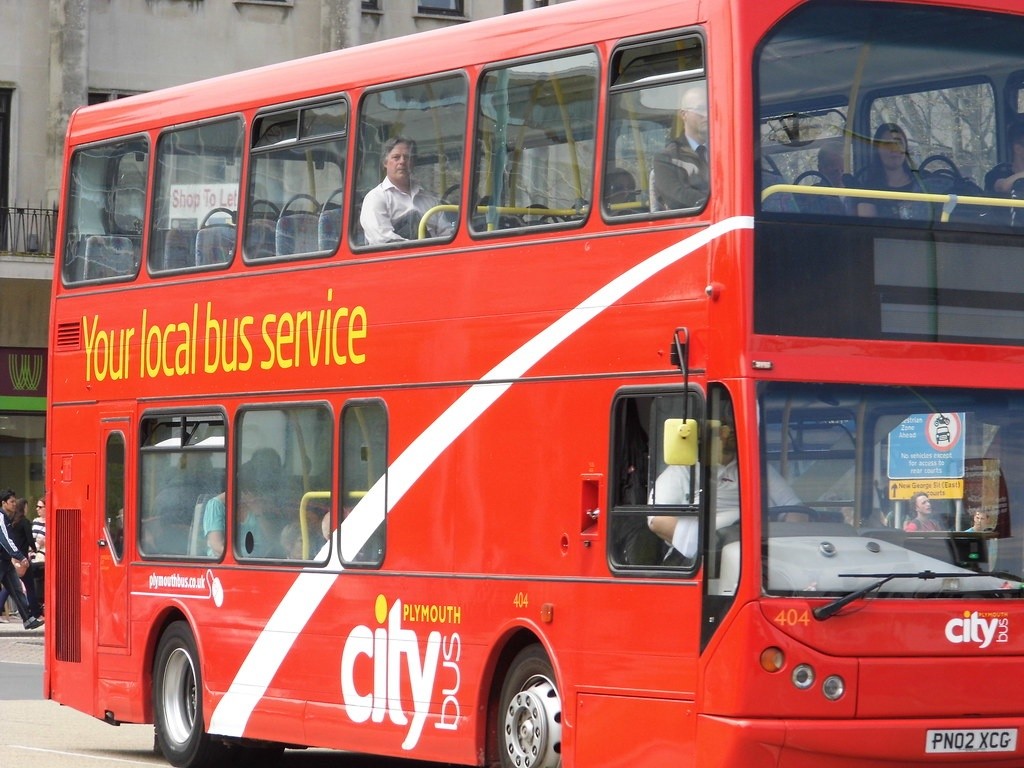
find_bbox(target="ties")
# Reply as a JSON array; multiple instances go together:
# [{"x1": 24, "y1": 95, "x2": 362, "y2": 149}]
[{"x1": 695, "y1": 146, "x2": 706, "y2": 167}]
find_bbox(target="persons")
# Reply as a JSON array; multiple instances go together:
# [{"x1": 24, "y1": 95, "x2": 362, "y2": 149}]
[
  {"x1": 879, "y1": 493, "x2": 993, "y2": 533},
  {"x1": 643, "y1": 390, "x2": 810, "y2": 559},
  {"x1": 984, "y1": 112, "x2": 1024, "y2": 225},
  {"x1": 359, "y1": 137, "x2": 452, "y2": 246},
  {"x1": 150, "y1": 452, "x2": 220, "y2": 556},
  {"x1": 602, "y1": 168, "x2": 637, "y2": 218},
  {"x1": 0, "y1": 490, "x2": 46, "y2": 630},
  {"x1": 795, "y1": 143, "x2": 854, "y2": 217},
  {"x1": 855, "y1": 123, "x2": 935, "y2": 223},
  {"x1": 205, "y1": 449, "x2": 320, "y2": 560},
  {"x1": 651, "y1": 86, "x2": 708, "y2": 211}
]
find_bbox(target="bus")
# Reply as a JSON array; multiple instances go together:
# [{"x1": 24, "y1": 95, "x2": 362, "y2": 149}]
[{"x1": 41, "y1": 0, "x2": 1024, "y2": 768}]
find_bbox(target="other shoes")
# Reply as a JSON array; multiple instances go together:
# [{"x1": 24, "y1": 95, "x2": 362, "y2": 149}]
[
  {"x1": 9, "y1": 610, "x2": 21, "y2": 619},
  {"x1": 0, "y1": 616, "x2": 10, "y2": 623}
]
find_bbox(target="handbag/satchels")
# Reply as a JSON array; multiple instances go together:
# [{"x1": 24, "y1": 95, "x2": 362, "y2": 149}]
[{"x1": 392, "y1": 209, "x2": 431, "y2": 239}]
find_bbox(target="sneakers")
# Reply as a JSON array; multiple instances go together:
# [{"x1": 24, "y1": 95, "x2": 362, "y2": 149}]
[{"x1": 23, "y1": 616, "x2": 44, "y2": 631}]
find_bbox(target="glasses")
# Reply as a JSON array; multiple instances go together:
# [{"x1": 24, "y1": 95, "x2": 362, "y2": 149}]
[{"x1": 36, "y1": 505, "x2": 45, "y2": 509}]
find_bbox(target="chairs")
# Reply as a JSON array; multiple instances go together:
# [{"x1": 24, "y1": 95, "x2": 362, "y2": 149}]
[
  {"x1": 81, "y1": 176, "x2": 568, "y2": 279},
  {"x1": 623, "y1": 436, "x2": 675, "y2": 569},
  {"x1": 649, "y1": 149, "x2": 1003, "y2": 225},
  {"x1": 190, "y1": 483, "x2": 369, "y2": 564}
]
